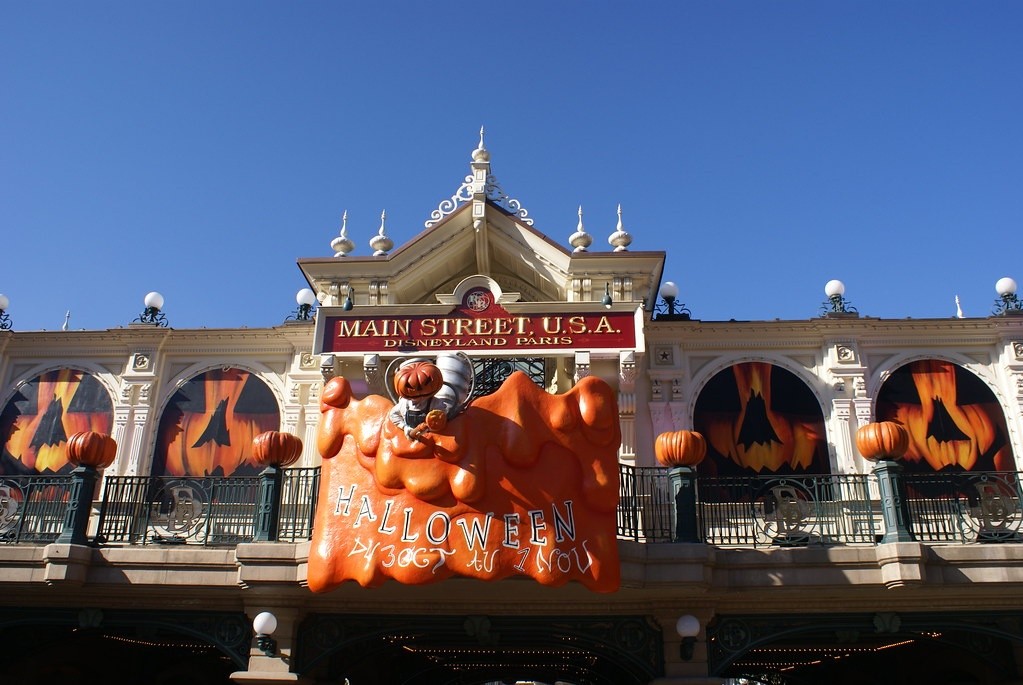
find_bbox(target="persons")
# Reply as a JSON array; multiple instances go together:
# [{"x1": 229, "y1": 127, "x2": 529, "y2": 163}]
[{"x1": 390, "y1": 396, "x2": 447, "y2": 440}]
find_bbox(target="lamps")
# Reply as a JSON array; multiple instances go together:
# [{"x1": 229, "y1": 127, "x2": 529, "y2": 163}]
[
  {"x1": 818, "y1": 280, "x2": 857, "y2": 317},
  {"x1": 132, "y1": 292, "x2": 169, "y2": 327},
  {"x1": 653, "y1": 281, "x2": 691, "y2": 320},
  {"x1": 676, "y1": 615, "x2": 700, "y2": 660},
  {"x1": 991, "y1": 277, "x2": 1023, "y2": 316},
  {"x1": 286, "y1": 288, "x2": 315, "y2": 321},
  {"x1": 603, "y1": 282, "x2": 612, "y2": 309},
  {"x1": 343, "y1": 288, "x2": 355, "y2": 310},
  {"x1": 0, "y1": 294, "x2": 12, "y2": 329},
  {"x1": 253, "y1": 612, "x2": 277, "y2": 657}
]
